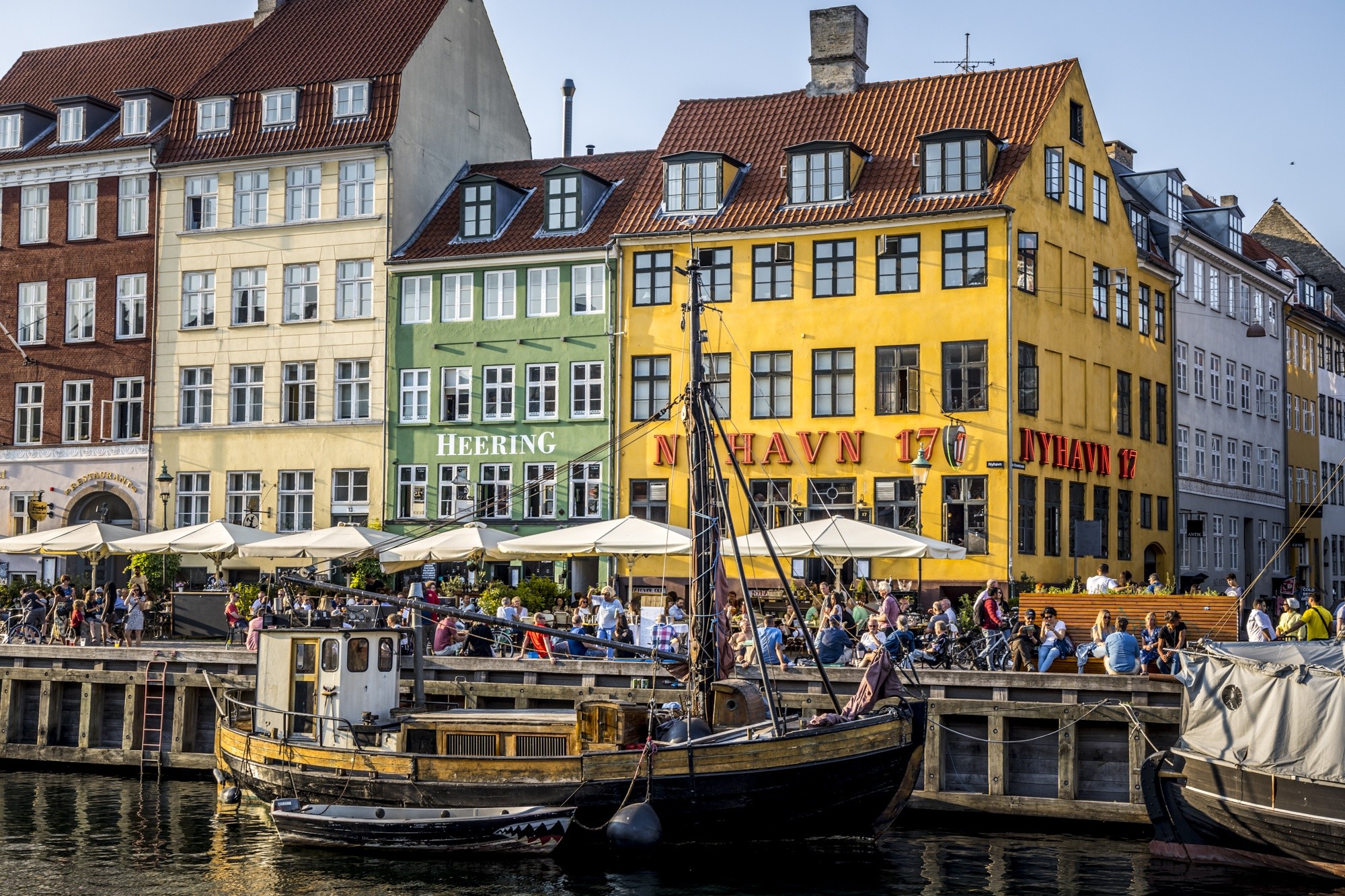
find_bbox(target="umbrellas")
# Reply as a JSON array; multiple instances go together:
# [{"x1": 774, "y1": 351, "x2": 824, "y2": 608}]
[
  {"x1": 717, "y1": 516, "x2": 968, "y2": 596},
  {"x1": 0, "y1": 522, "x2": 152, "y2": 591},
  {"x1": 379, "y1": 521, "x2": 524, "y2": 587},
  {"x1": 246, "y1": 521, "x2": 416, "y2": 607},
  {"x1": 109, "y1": 520, "x2": 287, "y2": 589},
  {"x1": 498, "y1": 516, "x2": 730, "y2": 559}
]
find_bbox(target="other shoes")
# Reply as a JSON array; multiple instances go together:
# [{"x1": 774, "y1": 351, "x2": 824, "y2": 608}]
[
  {"x1": 106, "y1": 637, "x2": 114, "y2": 644},
  {"x1": 42, "y1": 638, "x2": 48, "y2": 644},
  {"x1": 118, "y1": 638, "x2": 123, "y2": 646},
  {"x1": 1027, "y1": 663, "x2": 1035, "y2": 672},
  {"x1": 28, "y1": 639, "x2": 36, "y2": 644},
  {"x1": 91, "y1": 637, "x2": 97, "y2": 643}
]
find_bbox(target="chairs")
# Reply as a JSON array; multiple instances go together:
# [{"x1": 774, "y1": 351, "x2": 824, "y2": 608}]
[
  {"x1": 113, "y1": 611, "x2": 127, "y2": 638},
  {"x1": 860, "y1": 617, "x2": 870, "y2": 635},
  {"x1": 553, "y1": 611, "x2": 572, "y2": 632},
  {"x1": 224, "y1": 613, "x2": 244, "y2": 645},
  {"x1": 520, "y1": 616, "x2": 536, "y2": 652},
  {"x1": 890, "y1": 637, "x2": 912, "y2": 669},
  {"x1": 921, "y1": 639, "x2": 950, "y2": 669},
  {"x1": 844, "y1": 618, "x2": 864, "y2": 645},
  {"x1": 781, "y1": 617, "x2": 805, "y2": 655},
  {"x1": 142, "y1": 607, "x2": 158, "y2": 640},
  {"x1": 920, "y1": 623, "x2": 954, "y2": 647},
  {"x1": 543, "y1": 609, "x2": 554, "y2": 627}
]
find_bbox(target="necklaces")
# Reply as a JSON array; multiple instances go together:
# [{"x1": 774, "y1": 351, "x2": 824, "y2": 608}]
[{"x1": 1167, "y1": 625, "x2": 1176, "y2": 649}]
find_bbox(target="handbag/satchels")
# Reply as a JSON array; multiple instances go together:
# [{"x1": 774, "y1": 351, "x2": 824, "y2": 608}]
[
  {"x1": 1053, "y1": 634, "x2": 1075, "y2": 656},
  {"x1": 121, "y1": 615, "x2": 129, "y2": 623}
]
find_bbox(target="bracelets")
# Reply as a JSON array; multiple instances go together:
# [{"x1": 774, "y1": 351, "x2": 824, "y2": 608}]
[{"x1": 1151, "y1": 645, "x2": 1153, "y2": 648}]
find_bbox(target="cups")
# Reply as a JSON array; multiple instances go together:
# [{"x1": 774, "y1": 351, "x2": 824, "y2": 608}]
[
  {"x1": 115, "y1": 640, "x2": 120, "y2": 647},
  {"x1": 70, "y1": 640, "x2": 75, "y2": 646},
  {"x1": 65, "y1": 638, "x2": 70, "y2": 645},
  {"x1": 81, "y1": 638, "x2": 86, "y2": 646}
]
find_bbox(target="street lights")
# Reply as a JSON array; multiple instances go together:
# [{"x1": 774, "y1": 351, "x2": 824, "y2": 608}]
[
  {"x1": 154, "y1": 459, "x2": 173, "y2": 531},
  {"x1": 910, "y1": 441, "x2": 933, "y2": 614}
]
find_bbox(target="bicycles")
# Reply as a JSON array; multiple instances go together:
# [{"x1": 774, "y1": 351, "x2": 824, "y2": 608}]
[
  {"x1": 949, "y1": 624, "x2": 1014, "y2": 673},
  {"x1": 491, "y1": 627, "x2": 514, "y2": 658},
  {"x1": 0, "y1": 605, "x2": 42, "y2": 646}
]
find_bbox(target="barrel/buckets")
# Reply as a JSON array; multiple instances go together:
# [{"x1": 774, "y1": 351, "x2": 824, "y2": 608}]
[{"x1": 408, "y1": 582, "x2": 424, "y2": 601}]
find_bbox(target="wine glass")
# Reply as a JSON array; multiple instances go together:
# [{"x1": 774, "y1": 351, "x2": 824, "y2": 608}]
[{"x1": 341, "y1": 607, "x2": 347, "y2": 613}]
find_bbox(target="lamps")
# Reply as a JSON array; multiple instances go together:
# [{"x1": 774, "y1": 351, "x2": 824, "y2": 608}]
[{"x1": 1246, "y1": 318, "x2": 1268, "y2": 338}]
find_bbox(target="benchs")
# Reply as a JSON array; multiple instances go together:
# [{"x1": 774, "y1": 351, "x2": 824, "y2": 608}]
[{"x1": 1013, "y1": 589, "x2": 1245, "y2": 673}]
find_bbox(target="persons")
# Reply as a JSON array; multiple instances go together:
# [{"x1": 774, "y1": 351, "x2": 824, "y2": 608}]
[
  {"x1": 225, "y1": 571, "x2": 442, "y2": 656},
  {"x1": 1008, "y1": 606, "x2": 1067, "y2": 674},
  {"x1": 1090, "y1": 609, "x2": 1140, "y2": 677},
  {"x1": 973, "y1": 578, "x2": 1011, "y2": 672},
  {"x1": 1112, "y1": 570, "x2": 1166, "y2": 594},
  {"x1": 1223, "y1": 573, "x2": 1245, "y2": 632},
  {"x1": 1190, "y1": 584, "x2": 1202, "y2": 594},
  {"x1": 1139, "y1": 612, "x2": 1162, "y2": 676},
  {"x1": 0, "y1": 574, "x2": 170, "y2": 647},
  {"x1": 433, "y1": 586, "x2": 641, "y2": 665},
  {"x1": 208, "y1": 571, "x2": 227, "y2": 589},
  {"x1": 1086, "y1": 563, "x2": 1136, "y2": 594},
  {"x1": 649, "y1": 590, "x2": 794, "y2": 670},
  {"x1": 1243, "y1": 591, "x2": 1345, "y2": 642},
  {"x1": 128, "y1": 566, "x2": 149, "y2": 595},
  {"x1": 1157, "y1": 610, "x2": 1187, "y2": 675},
  {"x1": 784, "y1": 580, "x2": 958, "y2": 669}
]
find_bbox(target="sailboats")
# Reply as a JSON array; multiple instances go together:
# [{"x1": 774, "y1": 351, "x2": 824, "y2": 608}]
[
  {"x1": 1131, "y1": 453, "x2": 1345, "y2": 883},
  {"x1": 198, "y1": 224, "x2": 922, "y2": 859}
]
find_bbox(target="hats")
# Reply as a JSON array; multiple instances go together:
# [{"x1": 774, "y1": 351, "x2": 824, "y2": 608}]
[
  {"x1": 1024, "y1": 609, "x2": 1036, "y2": 618},
  {"x1": 830, "y1": 615, "x2": 841, "y2": 621},
  {"x1": 1286, "y1": 597, "x2": 1301, "y2": 610},
  {"x1": 96, "y1": 587, "x2": 104, "y2": 593}
]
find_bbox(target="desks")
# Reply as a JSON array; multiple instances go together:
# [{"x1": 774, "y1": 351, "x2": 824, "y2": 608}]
[
  {"x1": 794, "y1": 623, "x2": 819, "y2": 641},
  {"x1": 732, "y1": 624, "x2": 740, "y2": 629},
  {"x1": 908, "y1": 621, "x2": 929, "y2": 630},
  {"x1": 627, "y1": 619, "x2": 689, "y2": 657}
]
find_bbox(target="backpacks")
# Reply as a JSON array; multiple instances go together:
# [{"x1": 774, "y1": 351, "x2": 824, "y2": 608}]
[{"x1": 974, "y1": 598, "x2": 995, "y2": 626}]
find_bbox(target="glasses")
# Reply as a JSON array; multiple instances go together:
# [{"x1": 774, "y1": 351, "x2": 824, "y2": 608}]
[
  {"x1": 539, "y1": 617, "x2": 545, "y2": 620},
  {"x1": 235, "y1": 596, "x2": 240, "y2": 599},
  {"x1": 1026, "y1": 617, "x2": 1035, "y2": 620},
  {"x1": 601, "y1": 592, "x2": 608, "y2": 596},
  {"x1": 1044, "y1": 615, "x2": 1053, "y2": 620},
  {"x1": 305, "y1": 598, "x2": 311, "y2": 602},
  {"x1": 869, "y1": 625, "x2": 876, "y2": 628},
  {"x1": 133, "y1": 589, "x2": 139, "y2": 591},
  {"x1": 582, "y1": 601, "x2": 588, "y2": 604},
  {"x1": 1103, "y1": 616, "x2": 1111, "y2": 619},
  {"x1": 997, "y1": 591, "x2": 1003, "y2": 594},
  {"x1": 279, "y1": 594, "x2": 283, "y2": 596}
]
